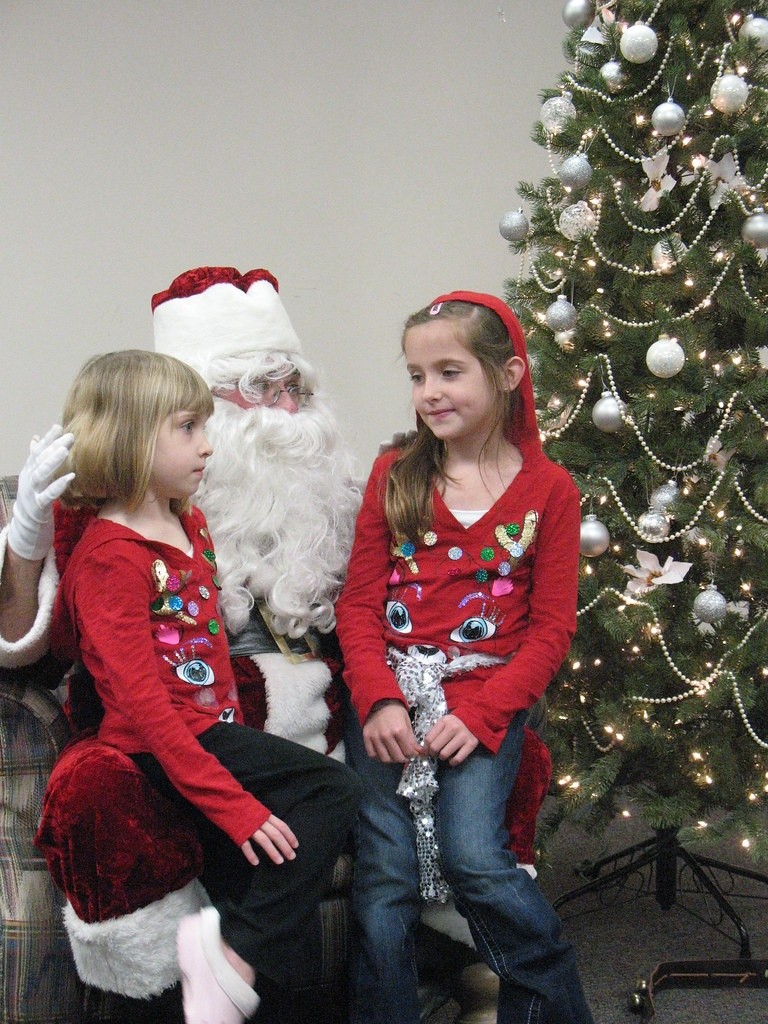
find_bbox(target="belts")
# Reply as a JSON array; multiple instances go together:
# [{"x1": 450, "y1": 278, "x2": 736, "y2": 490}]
[{"x1": 224, "y1": 600, "x2": 346, "y2": 664}]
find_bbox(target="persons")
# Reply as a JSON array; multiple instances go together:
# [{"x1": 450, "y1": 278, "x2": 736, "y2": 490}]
[
  {"x1": 0, "y1": 267, "x2": 556, "y2": 1024},
  {"x1": 335, "y1": 293, "x2": 601, "y2": 1024},
  {"x1": 57, "y1": 350, "x2": 366, "y2": 1023}
]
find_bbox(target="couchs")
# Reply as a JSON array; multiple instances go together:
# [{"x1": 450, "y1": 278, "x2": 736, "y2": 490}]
[{"x1": 0, "y1": 473, "x2": 550, "y2": 1024}]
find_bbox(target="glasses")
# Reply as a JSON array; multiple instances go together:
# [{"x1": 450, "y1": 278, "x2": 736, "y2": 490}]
[{"x1": 235, "y1": 379, "x2": 314, "y2": 410}]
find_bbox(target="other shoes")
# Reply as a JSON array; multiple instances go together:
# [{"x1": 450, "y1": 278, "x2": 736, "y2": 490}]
[{"x1": 175, "y1": 900, "x2": 262, "y2": 1024}]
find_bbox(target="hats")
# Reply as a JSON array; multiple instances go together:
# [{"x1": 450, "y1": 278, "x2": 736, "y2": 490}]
[{"x1": 152, "y1": 267, "x2": 304, "y2": 379}]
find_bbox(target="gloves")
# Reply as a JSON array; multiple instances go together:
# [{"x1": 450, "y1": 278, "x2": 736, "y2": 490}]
[{"x1": 8, "y1": 426, "x2": 80, "y2": 561}]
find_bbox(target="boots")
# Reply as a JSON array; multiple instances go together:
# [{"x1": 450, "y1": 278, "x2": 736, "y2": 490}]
[{"x1": 113, "y1": 976, "x2": 184, "y2": 1024}]
